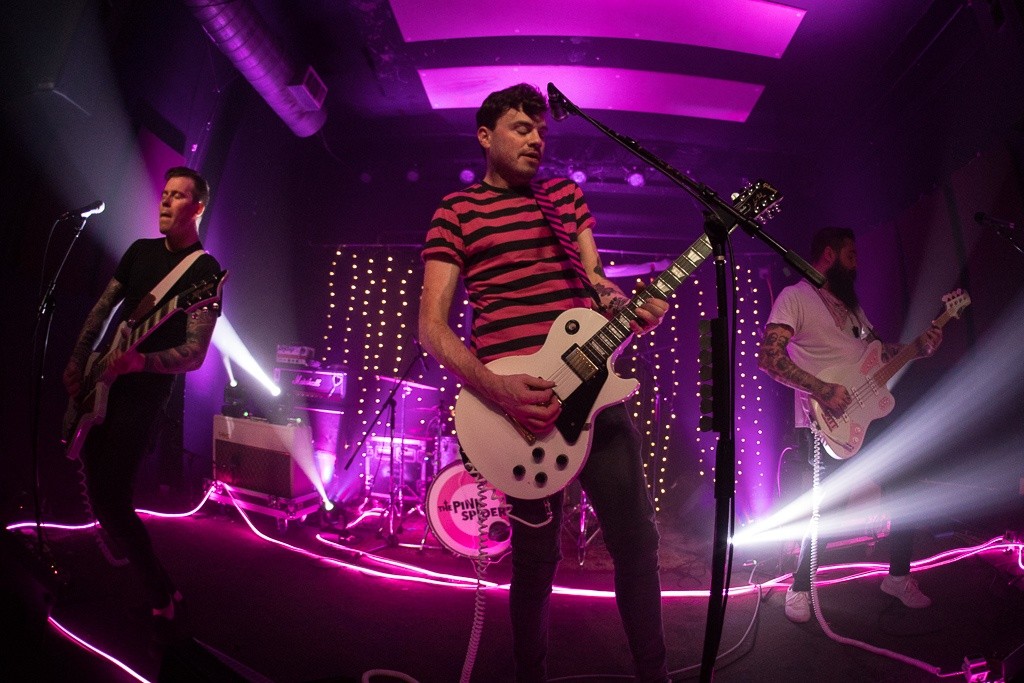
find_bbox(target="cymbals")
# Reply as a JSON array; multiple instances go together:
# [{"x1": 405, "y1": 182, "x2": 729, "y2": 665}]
[
  {"x1": 409, "y1": 404, "x2": 451, "y2": 414},
  {"x1": 378, "y1": 374, "x2": 439, "y2": 391}
]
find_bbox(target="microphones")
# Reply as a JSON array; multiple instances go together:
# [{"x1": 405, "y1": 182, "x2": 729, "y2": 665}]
[
  {"x1": 59, "y1": 200, "x2": 106, "y2": 222},
  {"x1": 413, "y1": 335, "x2": 429, "y2": 371},
  {"x1": 547, "y1": 82, "x2": 569, "y2": 121}
]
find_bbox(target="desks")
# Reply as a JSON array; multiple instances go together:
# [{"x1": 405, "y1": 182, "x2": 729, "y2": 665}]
[{"x1": 212, "y1": 412, "x2": 315, "y2": 497}]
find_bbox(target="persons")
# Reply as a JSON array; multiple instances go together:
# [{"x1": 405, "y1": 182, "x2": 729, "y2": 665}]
[
  {"x1": 758, "y1": 217, "x2": 946, "y2": 622},
  {"x1": 418, "y1": 83, "x2": 673, "y2": 683},
  {"x1": 63, "y1": 166, "x2": 228, "y2": 640}
]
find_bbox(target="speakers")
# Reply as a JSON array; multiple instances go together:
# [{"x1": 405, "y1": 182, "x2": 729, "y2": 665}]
[
  {"x1": 214, "y1": 415, "x2": 314, "y2": 512},
  {"x1": 277, "y1": 402, "x2": 348, "y2": 510}
]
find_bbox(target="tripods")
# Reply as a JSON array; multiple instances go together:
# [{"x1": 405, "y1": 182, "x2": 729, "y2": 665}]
[
  {"x1": 561, "y1": 486, "x2": 604, "y2": 566},
  {"x1": 344, "y1": 354, "x2": 448, "y2": 558}
]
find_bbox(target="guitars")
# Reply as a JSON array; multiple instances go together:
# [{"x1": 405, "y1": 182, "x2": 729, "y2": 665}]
[
  {"x1": 454, "y1": 179, "x2": 785, "y2": 500},
  {"x1": 61, "y1": 269, "x2": 230, "y2": 460},
  {"x1": 810, "y1": 288, "x2": 971, "y2": 460}
]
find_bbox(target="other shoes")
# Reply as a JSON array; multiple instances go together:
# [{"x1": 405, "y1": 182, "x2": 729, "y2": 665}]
[{"x1": 145, "y1": 580, "x2": 182, "y2": 656}]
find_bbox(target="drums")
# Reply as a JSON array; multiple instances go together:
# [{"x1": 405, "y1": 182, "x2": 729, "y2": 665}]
[
  {"x1": 424, "y1": 458, "x2": 513, "y2": 563},
  {"x1": 432, "y1": 435, "x2": 462, "y2": 478}
]
